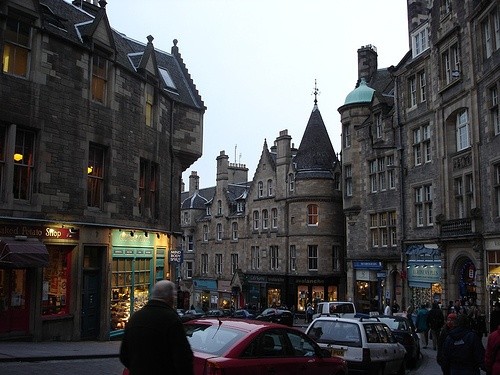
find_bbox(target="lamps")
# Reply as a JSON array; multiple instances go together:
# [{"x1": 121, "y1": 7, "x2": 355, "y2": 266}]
[
  {"x1": 68, "y1": 228, "x2": 78, "y2": 234},
  {"x1": 130, "y1": 230, "x2": 186, "y2": 242},
  {"x1": 405, "y1": 254, "x2": 435, "y2": 269}
]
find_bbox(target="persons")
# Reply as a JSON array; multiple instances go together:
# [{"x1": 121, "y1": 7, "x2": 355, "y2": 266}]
[
  {"x1": 191, "y1": 300, "x2": 500, "y2": 375},
  {"x1": 118, "y1": 280, "x2": 195, "y2": 375}
]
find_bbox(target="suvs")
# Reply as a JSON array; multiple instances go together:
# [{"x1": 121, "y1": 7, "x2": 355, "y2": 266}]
[{"x1": 312, "y1": 302, "x2": 357, "y2": 321}]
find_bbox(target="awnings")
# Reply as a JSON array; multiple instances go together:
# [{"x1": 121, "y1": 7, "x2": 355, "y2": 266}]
[{"x1": 0, "y1": 241, "x2": 49, "y2": 270}]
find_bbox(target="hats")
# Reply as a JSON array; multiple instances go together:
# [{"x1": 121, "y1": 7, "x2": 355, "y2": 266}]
[{"x1": 448, "y1": 314, "x2": 457, "y2": 320}]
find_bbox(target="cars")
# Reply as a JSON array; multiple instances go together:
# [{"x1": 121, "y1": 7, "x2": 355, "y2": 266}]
[
  {"x1": 255, "y1": 307, "x2": 294, "y2": 327},
  {"x1": 185, "y1": 310, "x2": 204, "y2": 315},
  {"x1": 208, "y1": 307, "x2": 254, "y2": 319},
  {"x1": 122, "y1": 315, "x2": 348, "y2": 375},
  {"x1": 305, "y1": 317, "x2": 408, "y2": 375},
  {"x1": 360, "y1": 315, "x2": 422, "y2": 371}
]
